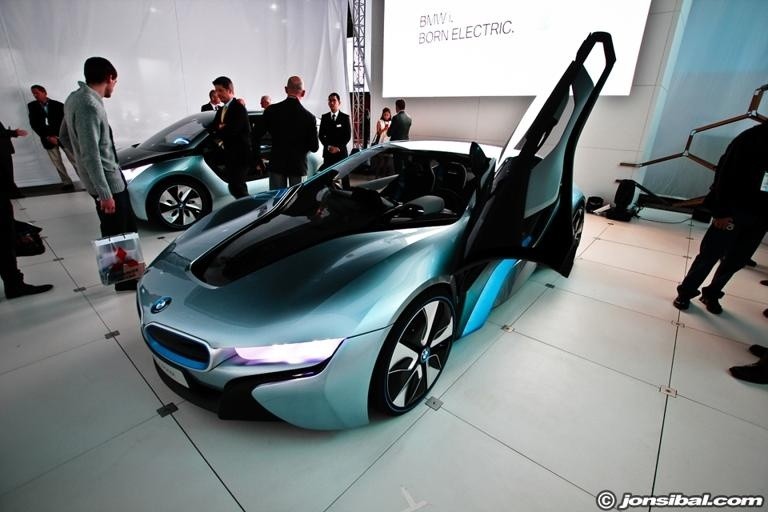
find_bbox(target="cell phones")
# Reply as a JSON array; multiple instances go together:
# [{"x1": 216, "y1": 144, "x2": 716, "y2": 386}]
[{"x1": 713, "y1": 219, "x2": 737, "y2": 231}]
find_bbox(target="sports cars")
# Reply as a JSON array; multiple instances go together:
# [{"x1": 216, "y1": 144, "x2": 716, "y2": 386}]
[
  {"x1": 144, "y1": 20, "x2": 619, "y2": 431},
  {"x1": 118, "y1": 107, "x2": 351, "y2": 231}
]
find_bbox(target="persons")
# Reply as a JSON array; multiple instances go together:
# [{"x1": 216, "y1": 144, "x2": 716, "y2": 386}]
[
  {"x1": 0, "y1": 85, "x2": 79, "y2": 299},
  {"x1": 201, "y1": 76, "x2": 413, "y2": 200},
  {"x1": 58, "y1": 56, "x2": 150, "y2": 290},
  {"x1": 674, "y1": 118, "x2": 768, "y2": 316},
  {"x1": 728, "y1": 345, "x2": 768, "y2": 384}
]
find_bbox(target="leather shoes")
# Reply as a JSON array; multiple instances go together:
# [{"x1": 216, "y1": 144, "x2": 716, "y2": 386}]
[
  {"x1": 729, "y1": 363, "x2": 768, "y2": 384},
  {"x1": 750, "y1": 344, "x2": 767, "y2": 358},
  {"x1": 4, "y1": 282, "x2": 53, "y2": 300},
  {"x1": 700, "y1": 296, "x2": 723, "y2": 314},
  {"x1": 673, "y1": 294, "x2": 690, "y2": 310}
]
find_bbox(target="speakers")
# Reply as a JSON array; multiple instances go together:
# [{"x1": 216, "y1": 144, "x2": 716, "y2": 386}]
[{"x1": 613, "y1": 179, "x2": 636, "y2": 212}]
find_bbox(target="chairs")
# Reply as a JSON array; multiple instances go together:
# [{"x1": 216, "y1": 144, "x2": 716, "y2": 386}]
[
  {"x1": 398, "y1": 157, "x2": 433, "y2": 205},
  {"x1": 428, "y1": 161, "x2": 470, "y2": 209}
]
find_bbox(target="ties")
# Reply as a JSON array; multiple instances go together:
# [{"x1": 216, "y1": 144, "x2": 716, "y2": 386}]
[
  {"x1": 332, "y1": 114, "x2": 335, "y2": 120},
  {"x1": 221, "y1": 105, "x2": 226, "y2": 123}
]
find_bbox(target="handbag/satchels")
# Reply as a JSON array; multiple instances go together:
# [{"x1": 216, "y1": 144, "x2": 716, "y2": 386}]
[
  {"x1": 14, "y1": 220, "x2": 45, "y2": 256},
  {"x1": 93, "y1": 212, "x2": 147, "y2": 286}
]
